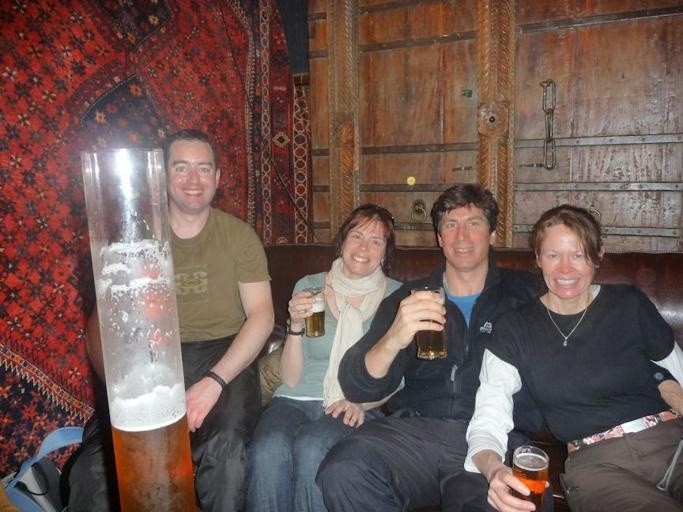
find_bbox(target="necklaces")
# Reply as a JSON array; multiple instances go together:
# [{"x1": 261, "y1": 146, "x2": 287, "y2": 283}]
[{"x1": 546, "y1": 289, "x2": 589, "y2": 348}]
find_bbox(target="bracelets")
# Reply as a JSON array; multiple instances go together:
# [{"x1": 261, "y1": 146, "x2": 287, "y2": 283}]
[
  {"x1": 288, "y1": 327, "x2": 305, "y2": 336},
  {"x1": 204, "y1": 371, "x2": 227, "y2": 389}
]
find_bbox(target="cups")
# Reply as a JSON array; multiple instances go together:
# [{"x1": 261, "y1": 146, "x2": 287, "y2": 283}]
[
  {"x1": 301, "y1": 286, "x2": 326, "y2": 338},
  {"x1": 409, "y1": 286, "x2": 449, "y2": 363},
  {"x1": 510, "y1": 444, "x2": 551, "y2": 512}
]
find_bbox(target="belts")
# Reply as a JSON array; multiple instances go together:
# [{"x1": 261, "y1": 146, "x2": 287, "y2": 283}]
[{"x1": 566, "y1": 410, "x2": 677, "y2": 453}]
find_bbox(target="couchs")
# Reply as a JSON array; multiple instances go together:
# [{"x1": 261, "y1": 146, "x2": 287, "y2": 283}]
[{"x1": 255, "y1": 242, "x2": 682, "y2": 512}]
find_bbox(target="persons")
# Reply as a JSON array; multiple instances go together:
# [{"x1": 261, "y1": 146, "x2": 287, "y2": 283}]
[
  {"x1": 245, "y1": 203, "x2": 403, "y2": 510},
  {"x1": 315, "y1": 184, "x2": 548, "y2": 511},
  {"x1": 464, "y1": 203, "x2": 682, "y2": 512},
  {"x1": 63, "y1": 128, "x2": 276, "y2": 511}
]
